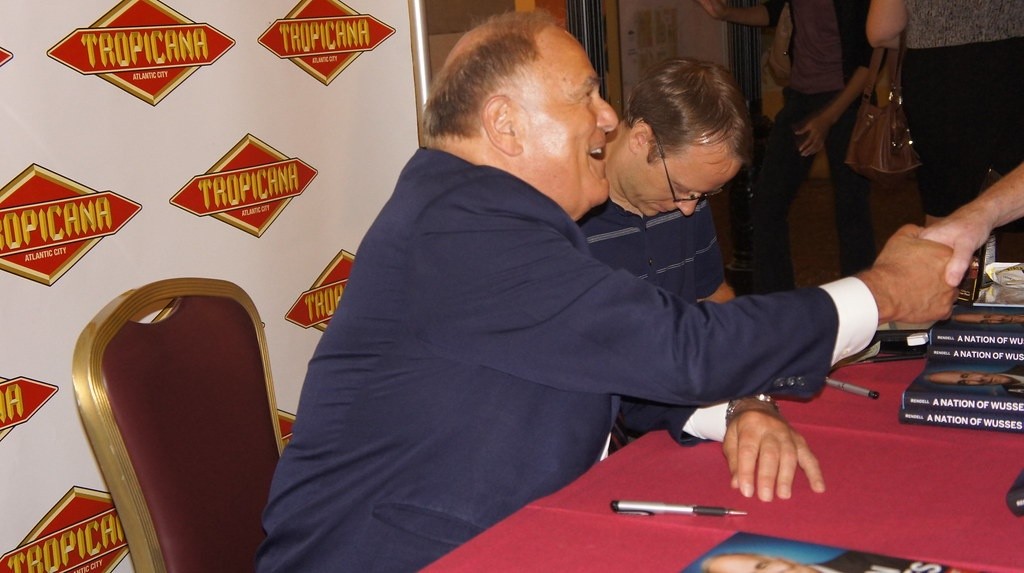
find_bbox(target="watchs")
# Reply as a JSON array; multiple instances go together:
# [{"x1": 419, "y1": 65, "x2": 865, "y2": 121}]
[{"x1": 725, "y1": 392, "x2": 782, "y2": 423}]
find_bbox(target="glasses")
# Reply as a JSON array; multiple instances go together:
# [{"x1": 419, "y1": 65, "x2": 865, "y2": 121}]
[{"x1": 637, "y1": 118, "x2": 722, "y2": 203}]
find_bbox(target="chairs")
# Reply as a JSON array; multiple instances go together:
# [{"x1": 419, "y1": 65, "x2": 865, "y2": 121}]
[{"x1": 70, "y1": 277, "x2": 284, "y2": 572}]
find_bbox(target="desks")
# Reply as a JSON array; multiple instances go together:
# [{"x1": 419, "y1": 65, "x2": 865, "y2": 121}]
[{"x1": 417, "y1": 353, "x2": 1024, "y2": 573}]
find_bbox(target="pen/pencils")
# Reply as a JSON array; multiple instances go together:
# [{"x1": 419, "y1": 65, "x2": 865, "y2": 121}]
[
  {"x1": 613, "y1": 497, "x2": 750, "y2": 520},
  {"x1": 823, "y1": 372, "x2": 881, "y2": 400}
]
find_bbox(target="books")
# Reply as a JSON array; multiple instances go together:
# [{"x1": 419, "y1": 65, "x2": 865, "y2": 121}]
[
  {"x1": 898, "y1": 301, "x2": 1024, "y2": 433},
  {"x1": 684, "y1": 530, "x2": 952, "y2": 573}
]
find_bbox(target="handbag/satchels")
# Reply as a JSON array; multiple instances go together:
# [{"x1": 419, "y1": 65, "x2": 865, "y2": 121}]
[
  {"x1": 845, "y1": 31, "x2": 923, "y2": 180},
  {"x1": 767, "y1": 1, "x2": 794, "y2": 78}
]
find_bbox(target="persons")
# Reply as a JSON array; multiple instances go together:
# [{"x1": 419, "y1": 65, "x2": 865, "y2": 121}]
[
  {"x1": 866, "y1": 1, "x2": 1024, "y2": 243},
  {"x1": 701, "y1": 550, "x2": 948, "y2": 573},
  {"x1": 698, "y1": 0, "x2": 891, "y2": 295},
  {"x1": 924, "y1": 363, "x2": 1024, "y2": 401},
  {"x1": 574, "y1": 58, "x2": 750, "y2": 304},
  {"x1": 952, "y1": 312, "x2": 1024, "y2": 328},
  {"x1": 919, "y1": 167, "x2": 1024, "y2": 288},
  {"x1": 249, "y1": 10, "x2": 957, "y2": 572}
]
